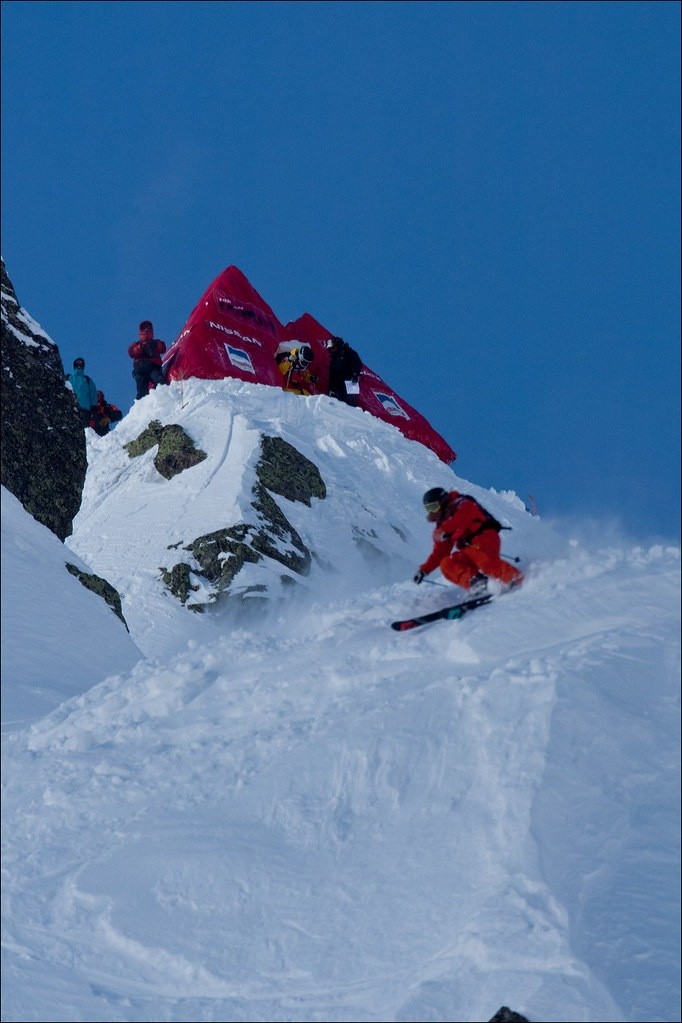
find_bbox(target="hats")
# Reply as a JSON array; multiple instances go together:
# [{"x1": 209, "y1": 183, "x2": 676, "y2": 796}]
[
  {"x1": 423, "y1": 487, "x2": 448, "y2": 504},
  {"x1": 302, "y1": 347, "x2": 314, "y2": 361}
]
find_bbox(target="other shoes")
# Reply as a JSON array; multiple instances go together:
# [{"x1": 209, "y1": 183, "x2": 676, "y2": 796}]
[
  {"x1": 464, "y1": 588, "x2": 487, "y2": 602},
  {"x1": 100, "y1": 418, "x2": 111, "y2": 427}
]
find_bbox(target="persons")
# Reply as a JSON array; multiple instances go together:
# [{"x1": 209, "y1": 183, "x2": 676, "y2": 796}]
[
  {"x1": 413, "y1": 488, "x2": 525, "y2": 600},
  {"x1": 91, "y1": 390, "x2": 123, "y2": 436},
  {"x1": 325, "y1": 337, "x2": 363, "y2": 405},
  {"x1": 128, "y1": 321, "x2": 166, "y2": 400},
  {"x1": 279, "y1": 340, "x2": 316, "y2": 396},
  {"x1": 66, "y1": 358, "x2": 97, "y2": 429}
]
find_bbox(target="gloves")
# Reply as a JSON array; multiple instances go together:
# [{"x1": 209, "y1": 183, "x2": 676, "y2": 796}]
[
  {"x1": 289, "y1": 354, "x2": 296, "y2": 361},
  {"x1": 413, "y1": 571, "x2": 423, "y2": 584},
  {"x1": 351, "y1": 373, "x2": 359, "y2": 385},
  {"x1": 310, "y1": 375, "x2": 319, "y2": 382},
  {"x1": 434, "y1": 531, "x2": 448, "y2": 541}
]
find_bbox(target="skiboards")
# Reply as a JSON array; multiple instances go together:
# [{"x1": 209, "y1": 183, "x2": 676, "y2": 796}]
[{"x1": 391, "y1": 584, "x2": 524, "y2": 631}]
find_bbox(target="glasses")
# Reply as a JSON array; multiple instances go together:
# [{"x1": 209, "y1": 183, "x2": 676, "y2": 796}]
[
  {"x1": 424, "y1": 501, "x2": 441, "y2": 512},
  {"x1": 300, "y1": 357, "x2": 310, "y2": 367}
]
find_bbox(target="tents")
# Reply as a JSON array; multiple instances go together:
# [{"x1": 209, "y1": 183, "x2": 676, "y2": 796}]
[{"x1": 149, "y1": 265, "x2": 455, "y2": 462}]
